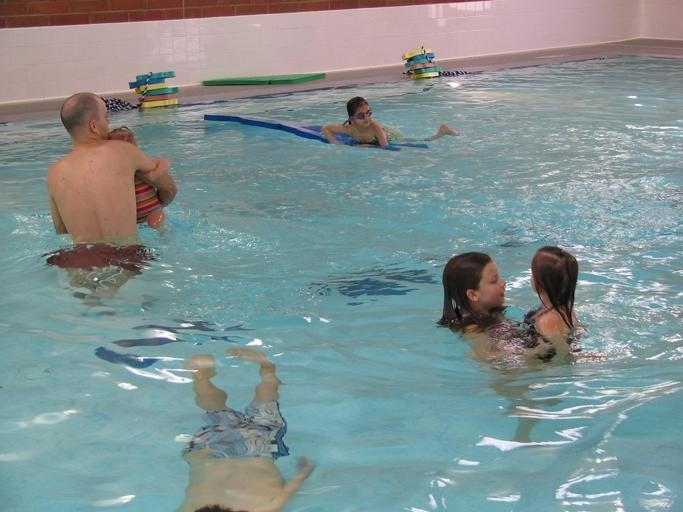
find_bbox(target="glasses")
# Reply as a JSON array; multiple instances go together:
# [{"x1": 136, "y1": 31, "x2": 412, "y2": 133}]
[{"x1": 355, "y1": 109, "x2": 374, "y2": 120}]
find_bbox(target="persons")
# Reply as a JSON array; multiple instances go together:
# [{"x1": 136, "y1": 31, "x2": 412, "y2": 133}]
[
  {"x1": 322, "y1": 96, "x2": 463, "y2": 148},
  {"x1": 434, "y1": 251, "x2": 552, "y2": 450},
  {"x1": 175, "y1": 347, "x2": 314, "y2": 511},
  {"x1": 107, "y1": 125, "x2": 170, "y2": 231},
  {"x1": 45, "y1": 91, "x2": 178, "y2": 311},
  {"x1": 523, "y1": 245, "x2": 583, "y2": 366}
]
